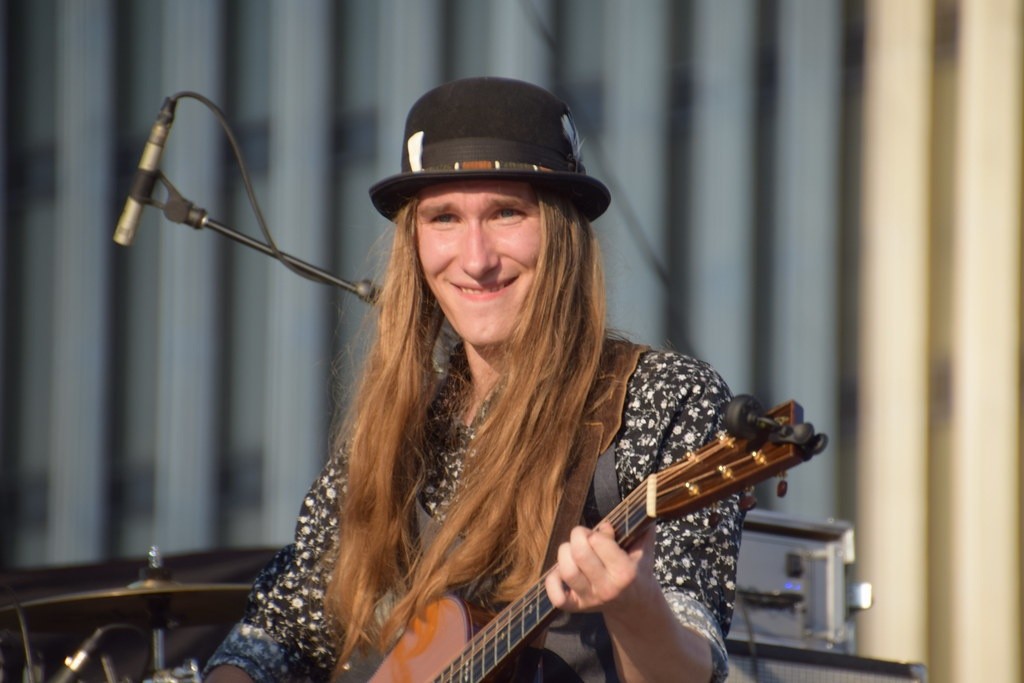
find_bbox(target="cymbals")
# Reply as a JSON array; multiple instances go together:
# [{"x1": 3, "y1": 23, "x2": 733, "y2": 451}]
[{"x1": 1, "y1": 579, "x2": 255, "y2": 642}]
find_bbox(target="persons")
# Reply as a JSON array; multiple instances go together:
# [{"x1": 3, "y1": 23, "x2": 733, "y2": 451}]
[{"x1": 202, "y1": 77, "x2": 746, "y2": 683}]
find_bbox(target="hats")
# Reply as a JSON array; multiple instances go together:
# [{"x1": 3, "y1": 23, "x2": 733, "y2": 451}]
[{"x1": 370, "y1": 77, "x2": 611, "y2": 223}]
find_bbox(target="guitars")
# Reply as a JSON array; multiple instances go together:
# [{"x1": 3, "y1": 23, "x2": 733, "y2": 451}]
[{"x1": 363, "y1": 397, "x2": 804, "y2": 683}]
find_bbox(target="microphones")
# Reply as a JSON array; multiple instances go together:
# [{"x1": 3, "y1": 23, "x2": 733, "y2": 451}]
[{"x1": 113, "y1": 101, "x2": 175, "y2": 248}]
